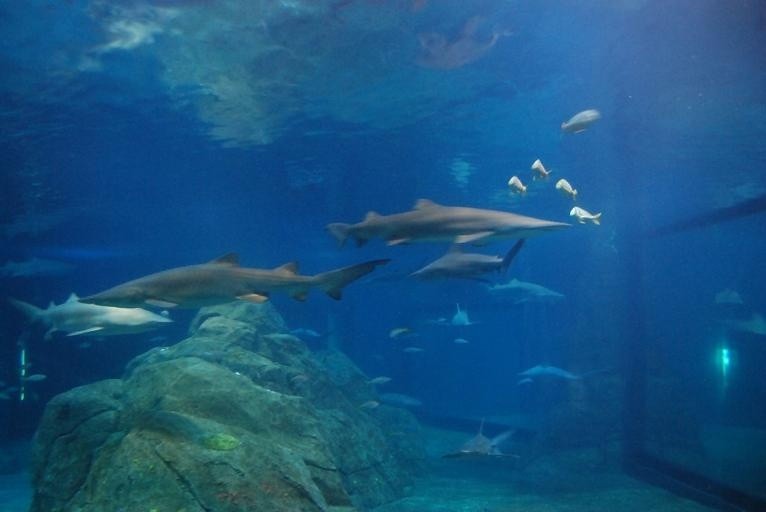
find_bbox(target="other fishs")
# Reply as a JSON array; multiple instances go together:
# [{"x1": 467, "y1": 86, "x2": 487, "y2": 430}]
[
  {"x1": 77, "y1": 255, "x2": 392, "y2": 311},
  {"x1": 9, "y1": 288, "x2": 177, "y2": 340}
]
